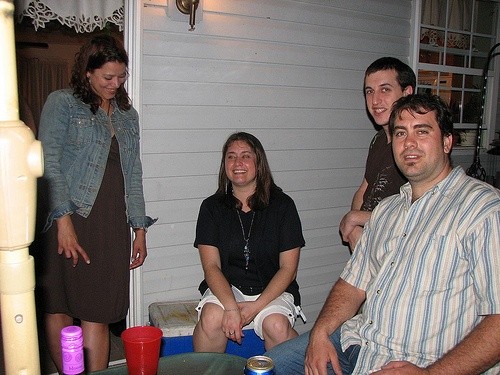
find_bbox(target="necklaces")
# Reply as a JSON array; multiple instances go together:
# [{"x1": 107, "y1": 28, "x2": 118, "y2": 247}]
[{"x1": 237, "y1": 210, "x2": 255, "y2": 269}]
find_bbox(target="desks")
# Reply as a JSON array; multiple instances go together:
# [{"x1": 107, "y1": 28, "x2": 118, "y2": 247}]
[{"x1": 90, "y1": 352, "x2": 246, "y2": 375}]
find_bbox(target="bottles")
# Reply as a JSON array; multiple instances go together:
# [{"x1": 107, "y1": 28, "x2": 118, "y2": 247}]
[{"x1": 61, "y1": 326, "x2": 86, "y2": 375}]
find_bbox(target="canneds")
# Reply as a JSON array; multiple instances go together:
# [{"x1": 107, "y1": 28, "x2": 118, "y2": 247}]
[{"x1": 244, "y1": 355, "x2": 276, "y2": 375}]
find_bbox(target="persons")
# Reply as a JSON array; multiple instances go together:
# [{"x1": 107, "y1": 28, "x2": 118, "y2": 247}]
[
  {"x1": 339, "y1": 57, "x2": 416, "y2": 253},
  {"x1": 265, "y1": 94, "x2": 500, "y2": 375},
  {"x1": 28, "y1": 35, "x2": 159, "y2": 375},
  {"x1": 193, "y1": 132, "x2": 306, "y2": 354}
]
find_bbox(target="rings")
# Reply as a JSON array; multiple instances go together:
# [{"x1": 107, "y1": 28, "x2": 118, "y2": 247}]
[
  {"x1": 230, "y1": 333, "x2": 234, "y2": 335},
  {"x1": 224, "y1": 332, "x2": 228, "y2": 333}
]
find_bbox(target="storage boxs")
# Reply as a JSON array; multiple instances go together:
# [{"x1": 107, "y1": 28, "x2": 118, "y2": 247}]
[{"x1": 148, "y1": 299, "x2": 266, "y2": 360}]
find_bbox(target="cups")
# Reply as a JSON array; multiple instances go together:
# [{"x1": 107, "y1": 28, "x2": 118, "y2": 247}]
[{"x1": 121, "y1": 326, "x2": 163, "y2": 375}]
